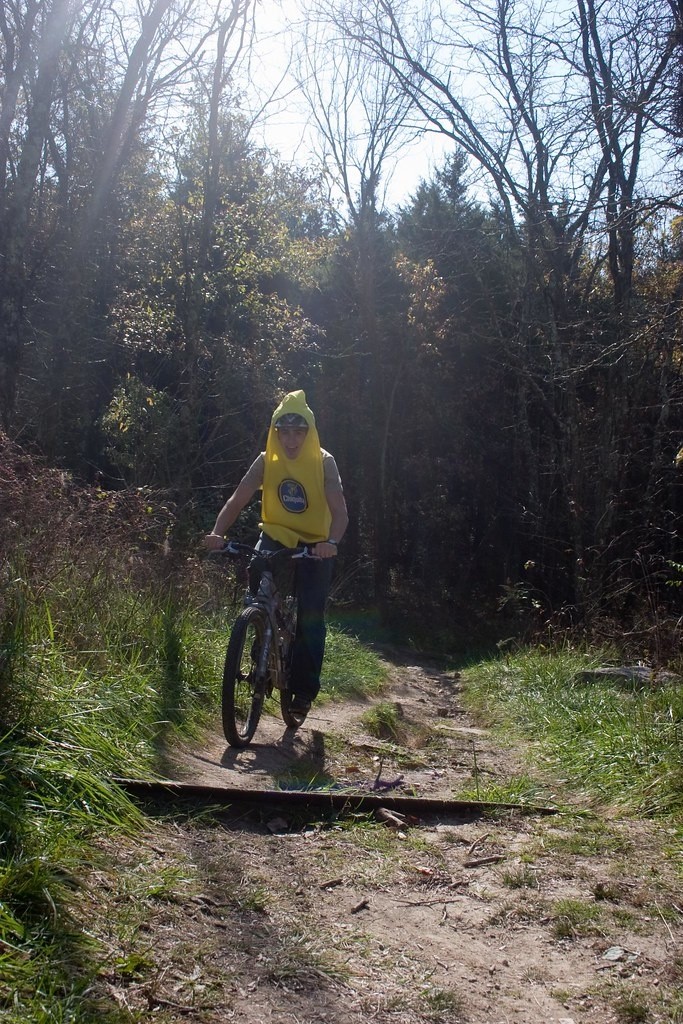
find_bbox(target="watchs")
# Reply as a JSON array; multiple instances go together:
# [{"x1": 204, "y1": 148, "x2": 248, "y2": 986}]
[{"x1": 327, "y1": 539, "x2": 337, "y2": 546}]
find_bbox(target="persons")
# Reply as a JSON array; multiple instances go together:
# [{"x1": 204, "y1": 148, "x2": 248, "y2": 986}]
[{"x1": 203, "y1": 389, "x2": 349, "y2": 716}]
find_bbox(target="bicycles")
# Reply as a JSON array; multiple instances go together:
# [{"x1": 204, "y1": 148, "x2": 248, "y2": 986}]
[{"x1": 209, "y1": 538, "x2": 321, "y2": 749}]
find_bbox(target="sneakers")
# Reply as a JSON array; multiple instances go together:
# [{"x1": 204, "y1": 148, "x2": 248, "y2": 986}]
[
  {"x1": 245, "y1": 673, "x2": 255, "y2": 684},
  {"x1": 288, "y1": 695, "x2": 311, "y2": 715}
]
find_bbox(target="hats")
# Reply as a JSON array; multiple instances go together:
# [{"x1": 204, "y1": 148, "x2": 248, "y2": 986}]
[{"x1": 274, "y1": 413, "x2": 308, "y2": 429}]
